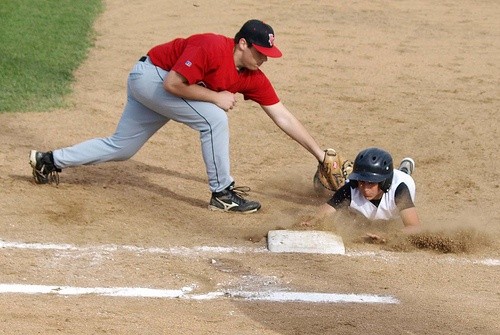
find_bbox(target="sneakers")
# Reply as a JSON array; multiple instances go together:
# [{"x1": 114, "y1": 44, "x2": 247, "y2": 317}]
[
  {"x1": 207, "y1": 181, "x2": 262, "y2": 215},
  {"x1": 28, "y1": 149, "x2": 62, "y2": 188},
  {"x1": 397, "y1": 157, "x2": 415, "y2": 177}
]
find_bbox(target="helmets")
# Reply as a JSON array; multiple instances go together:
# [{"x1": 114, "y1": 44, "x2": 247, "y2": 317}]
[{"x1": 348, "y1": 148, "x2": 394, "y2": 193}]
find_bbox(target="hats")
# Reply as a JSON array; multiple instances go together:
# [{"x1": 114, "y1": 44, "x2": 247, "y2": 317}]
[{"x1": 239, "y1": 18, "x2": 282, "y2": 58}]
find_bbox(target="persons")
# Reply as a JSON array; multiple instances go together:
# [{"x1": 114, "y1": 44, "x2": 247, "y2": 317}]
[
  {"x1": 29, "y1": 20, "x2": 353, "y2": 214},
  {"x1": 298, "y1": 148, "x2": 421, "y2": 244}
]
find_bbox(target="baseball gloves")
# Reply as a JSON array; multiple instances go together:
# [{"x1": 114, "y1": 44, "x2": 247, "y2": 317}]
[{"x1": 313, "y1": 148, "x2": 354, "y2": 200}]
